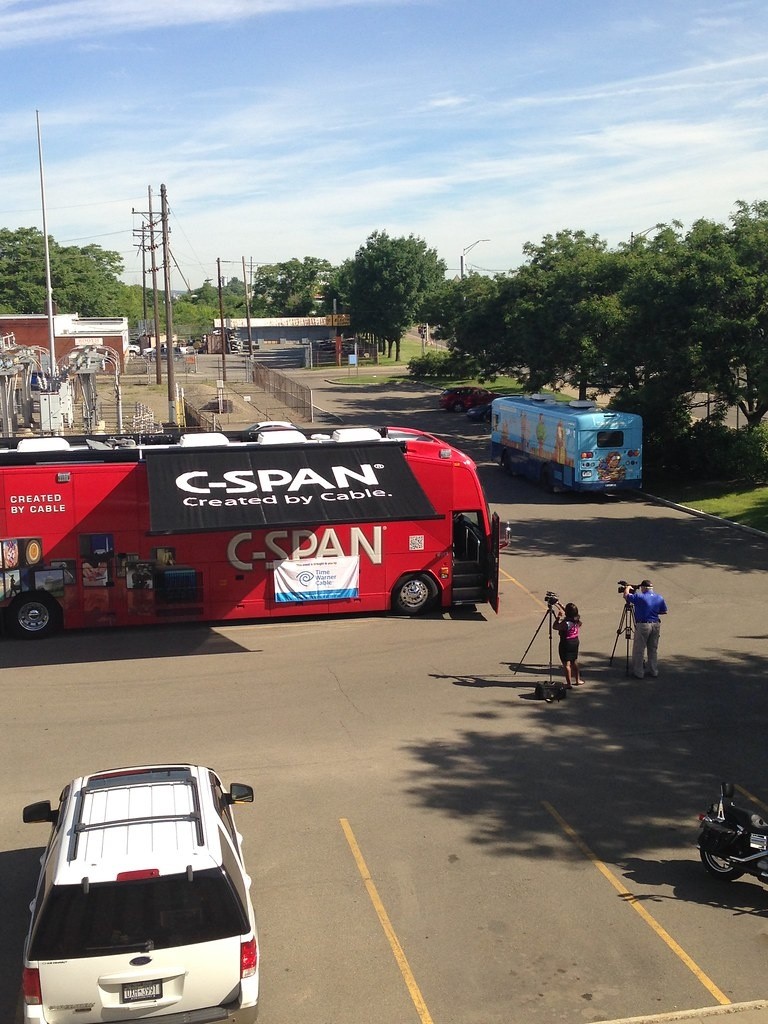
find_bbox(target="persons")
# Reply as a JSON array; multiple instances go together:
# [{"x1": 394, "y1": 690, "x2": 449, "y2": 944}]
[
  {"x1": 58, "y1": 547, "x2": 175, "y2": 589},
  {"x1": 623, "y1": 579, "x2": 668, "y2": 680},
  {"x1": 553, "y1": 603, "x2": 585, "y2": 689}
]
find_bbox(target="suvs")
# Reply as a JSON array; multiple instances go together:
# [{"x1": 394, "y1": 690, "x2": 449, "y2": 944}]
[
  {"x1": 150, "y1": 347, "x2": 183, "y2": 362},
  {"x1": 439, "y1": 385, "x2": 505, "y2": 413},
  {"x1": 20, "y1": 762, "x2": 265, "y2": 1023}
]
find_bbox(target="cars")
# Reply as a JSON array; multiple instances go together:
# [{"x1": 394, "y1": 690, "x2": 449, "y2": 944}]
[
  {"x1": 129, "y1": 345, "x2": 140, "y2": 357},
  {"x1": 466, "y1": 402, "x2": 492, "y2": 423},
  {"x1": 185, "y1": 345, "x2": 203, "y2": 354}
]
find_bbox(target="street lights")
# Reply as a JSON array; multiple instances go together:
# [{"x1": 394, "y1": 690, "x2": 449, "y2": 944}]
[
  {"x1": 458, "y1": 239, "x2": 491, "y2": 279},
  {"x1": 630, "y1": 221, "x2": 659, "y2": 253}
]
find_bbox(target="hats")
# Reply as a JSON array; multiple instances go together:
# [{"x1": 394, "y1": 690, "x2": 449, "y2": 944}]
[{"x1": 638, "y1": 580, "x2": 653, "y2": 587}]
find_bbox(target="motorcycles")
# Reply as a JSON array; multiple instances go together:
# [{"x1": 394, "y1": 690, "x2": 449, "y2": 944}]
[{"x1": 697, "y1": 780, "x2": 768, "y2": 891}]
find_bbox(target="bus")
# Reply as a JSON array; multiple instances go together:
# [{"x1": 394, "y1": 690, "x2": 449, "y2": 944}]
[
  {"x1": 1, "y1": 425, "x2": 511, "y2": 628},
  {"x1": 490, "y1": 392, "x2": 643, "y2": 495}
]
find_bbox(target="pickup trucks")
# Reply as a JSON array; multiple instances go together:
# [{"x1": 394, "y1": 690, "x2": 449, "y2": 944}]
[{"x1": 142, "y1": 343, "x2": 186, "y2": 358}]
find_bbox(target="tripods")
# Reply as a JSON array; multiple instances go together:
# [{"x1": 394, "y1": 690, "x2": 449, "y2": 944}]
[
  {"x1": 609, "y1": 600, "x2": 636, "y2": 676},
  {"x1": 514, "y1": 604, "x2": 557, "y2": 684}
]
